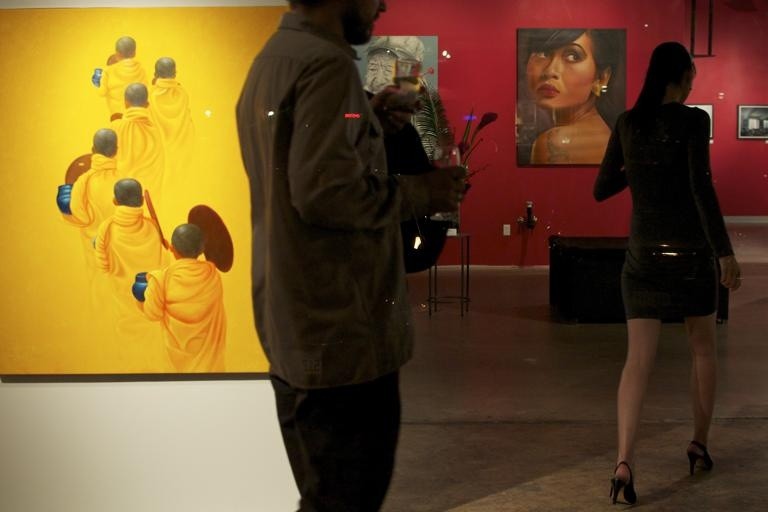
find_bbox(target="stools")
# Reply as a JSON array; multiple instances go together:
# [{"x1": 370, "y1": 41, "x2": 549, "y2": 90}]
[{"x1": 426, "y1": 234, "x2": 470, "y2": 317}]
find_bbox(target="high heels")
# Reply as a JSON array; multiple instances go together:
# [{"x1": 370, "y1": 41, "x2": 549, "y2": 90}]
[
  {"x1": 687, "y1": 440, "x2": 713, "y2": 474},
  {"x1": 609, "y1": 461, "x2": 637, "y2": 505}
]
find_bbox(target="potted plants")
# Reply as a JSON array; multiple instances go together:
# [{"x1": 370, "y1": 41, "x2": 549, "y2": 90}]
[{"x1": 417, "y1": 80, "x2": 497, "y2": 235}]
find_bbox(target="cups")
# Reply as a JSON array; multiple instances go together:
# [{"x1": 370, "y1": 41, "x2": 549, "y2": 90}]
[{"x1": 394, "y1": 58, "x2": 421, "y2": 128}]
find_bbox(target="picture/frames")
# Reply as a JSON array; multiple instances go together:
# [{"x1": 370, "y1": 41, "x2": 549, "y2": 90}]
[
  {"x1": 737, "y1": 105, "x2": 768, "y2": 139},
  {"x1": 687, "y1": 104, "x2": 713, "y2": 138}
]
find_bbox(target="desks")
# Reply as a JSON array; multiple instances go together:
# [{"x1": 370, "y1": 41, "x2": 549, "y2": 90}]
[{"x1": 548, "y1": 234, "x2": 731, "y2": 323}]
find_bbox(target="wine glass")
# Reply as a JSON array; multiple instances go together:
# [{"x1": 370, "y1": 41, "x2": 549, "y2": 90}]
[{"x1": 430, "y1": 146, "x2": 461, "y2": 223}]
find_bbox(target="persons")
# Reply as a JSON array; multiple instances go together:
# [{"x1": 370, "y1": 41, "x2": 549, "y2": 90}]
[
  {"x1": 370, "y1": 87, "x2": 436, "y2": 179},
  {"x1": 360, "y1": 36, "x2": 426, "y2": 93},
  {"x1": 233, "y1": 1, "x2": 474, "y2": 511},
  {"x1": 517, "y1": 29, "x2": 619, "y2": 166},
  {"x1": 589, "y1": 39, "x2": 746, "y2": 505}
]
